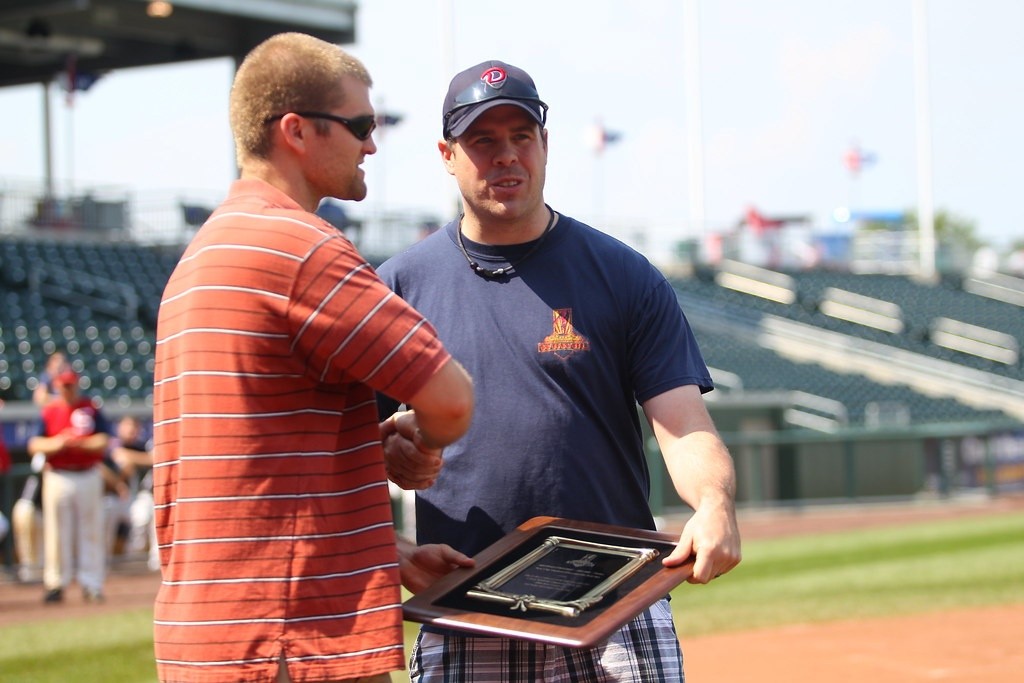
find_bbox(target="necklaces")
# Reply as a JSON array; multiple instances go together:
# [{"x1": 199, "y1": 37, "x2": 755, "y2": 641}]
[{"x1": 456, "y1": 203, "x2": 555, "y2": 282}]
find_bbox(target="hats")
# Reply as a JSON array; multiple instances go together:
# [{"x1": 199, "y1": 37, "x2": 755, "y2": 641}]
[
  {"x1": 442, "y1": 60, "x2": 549, "y2": 137},
  {"x1": 58, "y1": 368, "x2": 79, "y2": 386}
]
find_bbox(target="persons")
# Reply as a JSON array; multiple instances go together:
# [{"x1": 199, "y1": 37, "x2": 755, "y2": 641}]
[
  {"x1": 0, "y1": 351, "x2": 162, "y2": 602},
  {"x1": 355, "y1": 61, "x2": 743, "y2": 683},
  {"x1": 151, "y1": 32, "x2": 475, "y2": 683}
]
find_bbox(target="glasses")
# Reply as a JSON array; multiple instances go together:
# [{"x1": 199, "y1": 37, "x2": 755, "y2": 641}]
[
  {"x1": 443, "y1": 73, "x2": 548, "y2": 139},
  {"x1": 264, "y1": 109, "x2": 377, "y2": 141}
]
